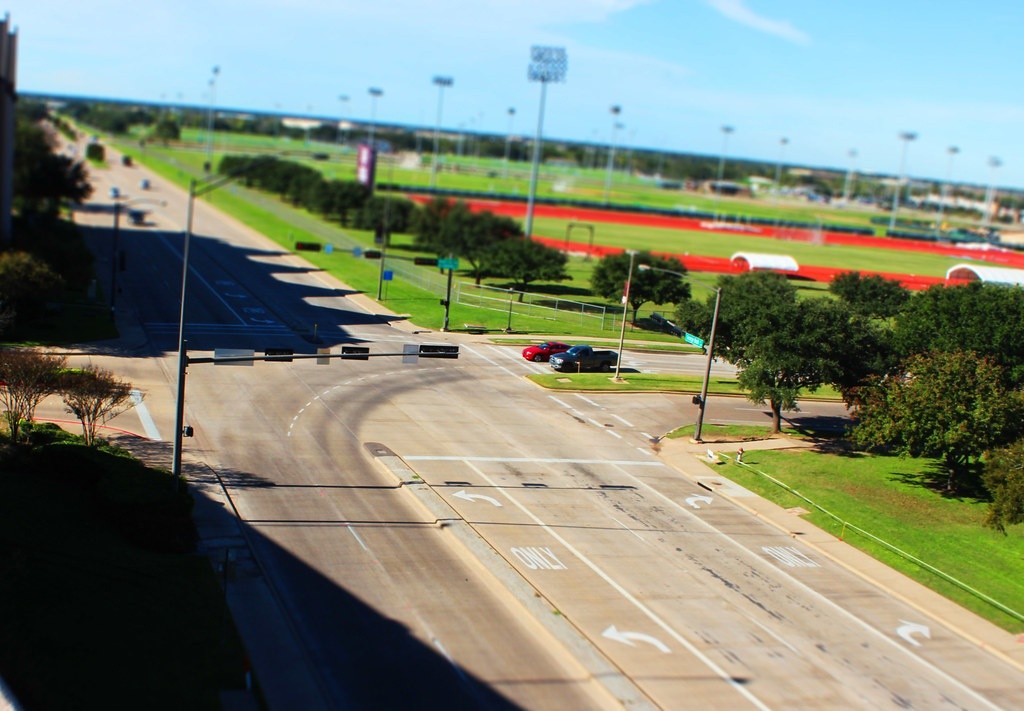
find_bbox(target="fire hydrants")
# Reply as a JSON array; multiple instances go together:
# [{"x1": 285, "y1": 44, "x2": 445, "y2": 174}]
[{"x1": 736, "y1": 447, "x2": 744, "y2": 462}]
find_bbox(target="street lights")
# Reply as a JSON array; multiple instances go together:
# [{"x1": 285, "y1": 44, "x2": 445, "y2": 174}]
[
  {"x1": 614, "y1": 248, "x2": 639, "y2": 379},
  {"x1": 637, "y1": 264, "x2": 724, "y2": 443},
  {"x1": 172, "y1": 148, "x2": 329, "y2": 476},
  {"x1": 111, "y1": 197, "x2": 167, "y2": 314}
]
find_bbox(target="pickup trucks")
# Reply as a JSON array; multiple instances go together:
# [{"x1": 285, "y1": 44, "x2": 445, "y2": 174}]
[
  {"x1": 522, "y1": 341, "x2": 573, "y2": 363},
  {"x1": 549, "y1": 344, "x2": 618, "y2": 373}
]
